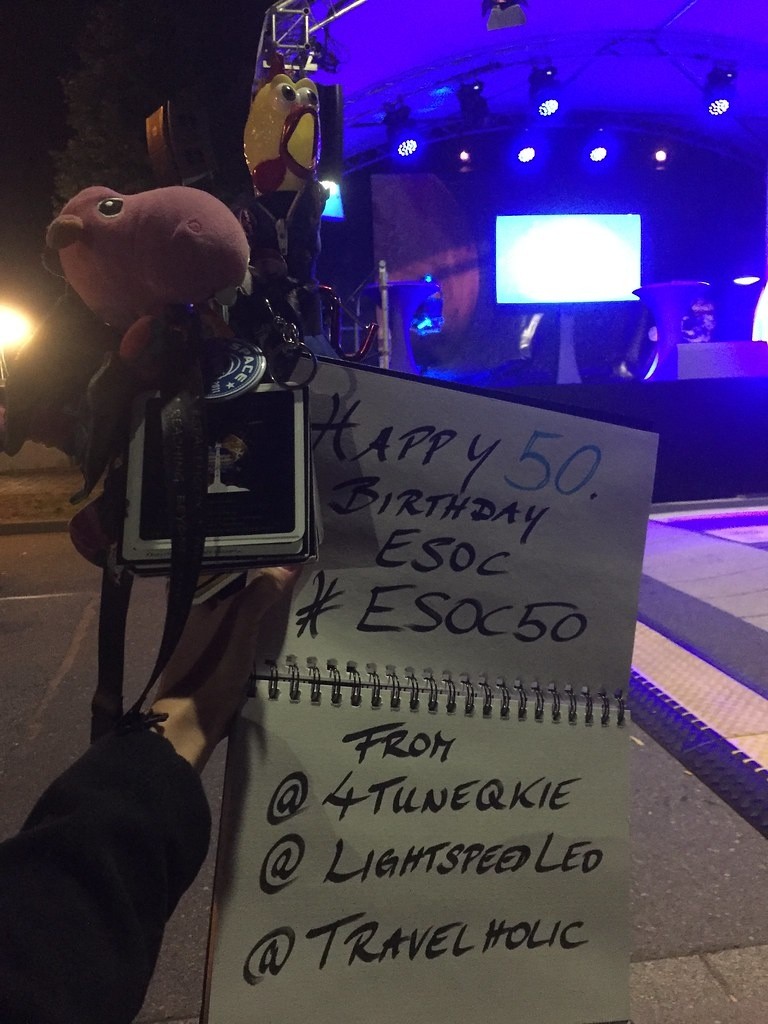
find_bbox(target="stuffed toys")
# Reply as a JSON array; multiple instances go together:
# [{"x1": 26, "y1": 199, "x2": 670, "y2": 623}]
[{"x1": 0, "y1": 183, "x2": 250, "y2": 560}]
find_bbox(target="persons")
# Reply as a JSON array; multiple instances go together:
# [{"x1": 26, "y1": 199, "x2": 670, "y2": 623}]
[{"x1": 0, "y1": 565, "x2": 294, "y2": 1024}]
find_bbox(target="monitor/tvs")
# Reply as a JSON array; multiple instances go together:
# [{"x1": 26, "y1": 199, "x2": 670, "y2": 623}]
[{"x1": 496, "y1": 213, "x2": 642, "y2": 303}]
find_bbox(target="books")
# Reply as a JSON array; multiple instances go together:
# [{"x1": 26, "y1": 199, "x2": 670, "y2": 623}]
[{"x1": 122, "y1": 352, "x2": 661, "y2": 1024}]
